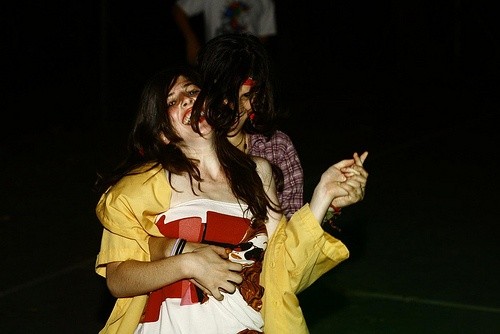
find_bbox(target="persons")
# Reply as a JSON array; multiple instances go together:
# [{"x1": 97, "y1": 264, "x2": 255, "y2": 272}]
[
  {"x1": 140, "y1": 30, "x2": 370, "y2": 333},
  {"x1": 95, "y1": 63, "x2": 369, "y2": 334},
  {"x1": 172, "y1": 1, "x2": 278, "y2": 66}
]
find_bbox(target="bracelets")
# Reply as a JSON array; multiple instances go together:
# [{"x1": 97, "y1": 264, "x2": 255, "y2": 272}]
[
  {"x1": 170, "y1": 237, "x2": 181, "y2": 256},
  {"x1": 324, "y1": 211, "x2": 333, "y2": 222},
  {"x1": 174, "y1": 238, "x2": 185, "y2": 256},
  {"x1": 328, "y1": 202, "x2": 342, "y2": 215},
  {"x1": 180, "y1": 240, "x2": 188, "y2": 258}
]
